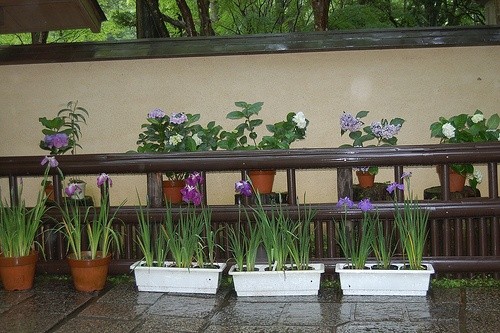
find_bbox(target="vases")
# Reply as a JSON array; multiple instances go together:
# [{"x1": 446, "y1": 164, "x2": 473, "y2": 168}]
[
  {"x1": 436, "y1": 165, "x2": 466, "y2": 192},
  {"x1": 335, "y1": 262, "x2": 435, "y2": 296},
  {"x1": 129, "y1": 261, "x2": 227, "y2": 294},
  {"x1": 228, "y1": 263, "x2": 325, "y2": 296},
  {"x1": 67, "y1": 251, "x2": 112, "y2": 293},
  {"x1": 247, "y1": 168, "x2": 276, "y2": 194},
  {"x1": 162, "y1": 181, "x2": 184, "y2": 204},
  {"x1": 0, "y1": 249, "x2": 38, "y2": 292},
  {"x1": 356, "y1": 170, "x2": 375, "y2": 188}
]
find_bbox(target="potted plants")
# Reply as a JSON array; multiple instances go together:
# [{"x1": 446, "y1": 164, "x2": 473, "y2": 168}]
[{"x1": 44, "y1": 179, "x2": 87, "y2": 201}]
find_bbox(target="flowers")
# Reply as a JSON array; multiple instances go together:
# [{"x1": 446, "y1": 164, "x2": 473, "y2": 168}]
[
  {"x1": 321, "y1": 170, "x2": 431, "y2": 270},
  {"x1": 36, "y1": 166, "x2": 128, "y2": 260},
  {"x1": 340, "y1": 108, "x2": 405, "y2": 175},
  {"x1": 222, "y1": 174, "x2": 318, "y2": 272},
  {"x1": 220, "y1": 100, "x2": 310, "y2": 151},
  {"x1": 430, "y1": 109, "x2": 500, "y2": 191},
  {"x1": 127, "y1": 109, "x2": 222, "y2": 180},
  {"x1": 0, "y1": 100, "x2": 89, "y2": 258},
  {"x1": 129, "y1": 170, "x2": 225, "y2": 273}
]
